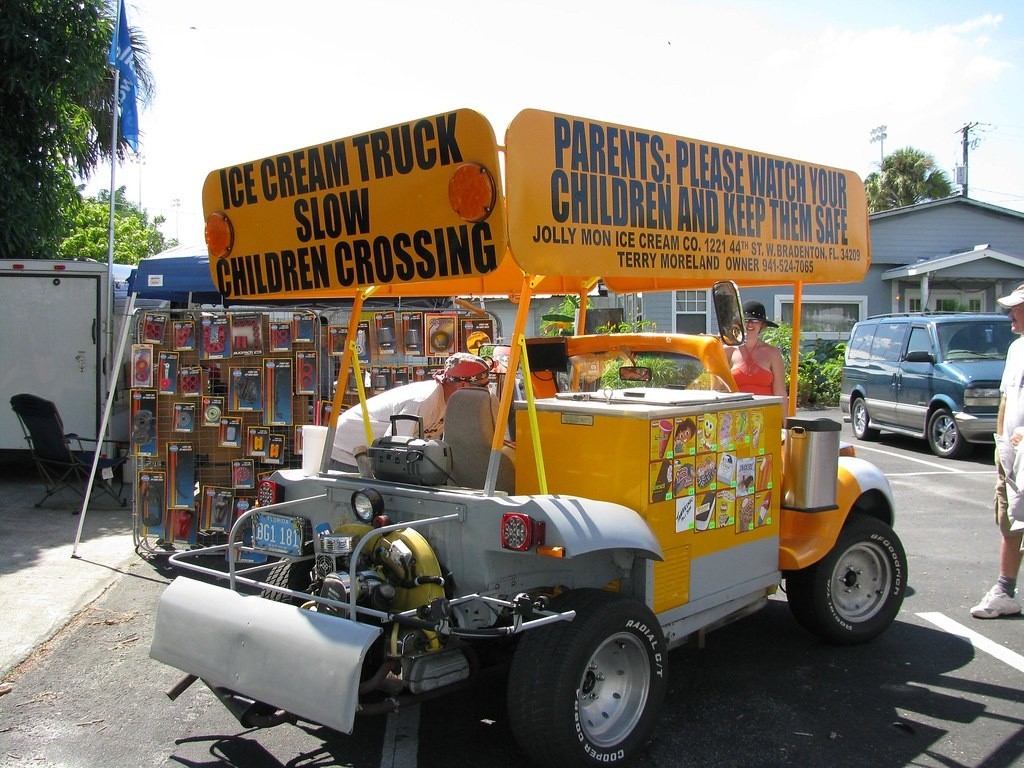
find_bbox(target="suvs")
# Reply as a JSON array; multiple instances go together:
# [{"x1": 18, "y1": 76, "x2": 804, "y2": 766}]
[{"x1": 840, "y1": 310, "x2": 1020, "y2": 458}]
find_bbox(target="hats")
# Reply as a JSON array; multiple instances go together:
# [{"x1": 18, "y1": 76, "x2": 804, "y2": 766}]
[
  {"x1": 997, "y1": 290, "x2": 1024, "y2": 309},
  {"x1": 493, "y1": 346, "x2": 510, "y2": 361},
  {"x1": 433, "y1": 352, "x2": 490, "y2": 384},
  {"x1": 743, "y1": 301, "x2": 779, "y2": 328}
]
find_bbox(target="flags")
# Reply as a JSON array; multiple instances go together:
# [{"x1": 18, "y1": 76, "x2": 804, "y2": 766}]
[{"x1": 108, "y1": 0, "x2": 139, "y2": 154}]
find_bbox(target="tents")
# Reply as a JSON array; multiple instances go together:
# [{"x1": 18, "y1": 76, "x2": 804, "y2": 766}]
[{"x1": 74, "y1": 239, "x2": 488, "y2": 559}]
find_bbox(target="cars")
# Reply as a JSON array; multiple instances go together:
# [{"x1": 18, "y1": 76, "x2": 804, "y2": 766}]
[{"x1": 152, "y1": 106, "x2": 909, "y2": 767}]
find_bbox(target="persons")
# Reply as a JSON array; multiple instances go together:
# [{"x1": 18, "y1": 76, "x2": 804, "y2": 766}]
[
  {"x1": 710, "y1": 300, "x2": 789, "y2": 432},
  {"x1": 970, "y1": 284, "x2": 1024, "y2": 619},
  {"x1": 332, "y1": 352, "x2": 491, "y2": 473}
]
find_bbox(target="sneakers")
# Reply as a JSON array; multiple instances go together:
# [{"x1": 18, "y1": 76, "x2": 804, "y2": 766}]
[{"x1": 970, "y1": 584, "x2": 1021, "y2": 617}]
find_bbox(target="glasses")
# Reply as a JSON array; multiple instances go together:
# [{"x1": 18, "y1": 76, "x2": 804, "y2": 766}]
[
  {"x1": 468, "y1": 380, "x2": 491, "y2": 387},
  {"x1": 745, "y1": 319, "x2": 760, "y2": 323}
]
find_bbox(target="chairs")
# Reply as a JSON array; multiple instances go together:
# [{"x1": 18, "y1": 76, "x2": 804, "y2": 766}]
[
  {"x1": 9, "y1": 394, "x2": 131, "y2": 517},
  {"x1": 442, "y1": 386, "x2": 517, "y2": 496}
]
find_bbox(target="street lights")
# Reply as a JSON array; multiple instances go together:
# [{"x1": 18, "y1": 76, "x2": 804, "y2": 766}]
[{"x1": 869, "y1": 125, "x2": 887, "y2": 162}]
